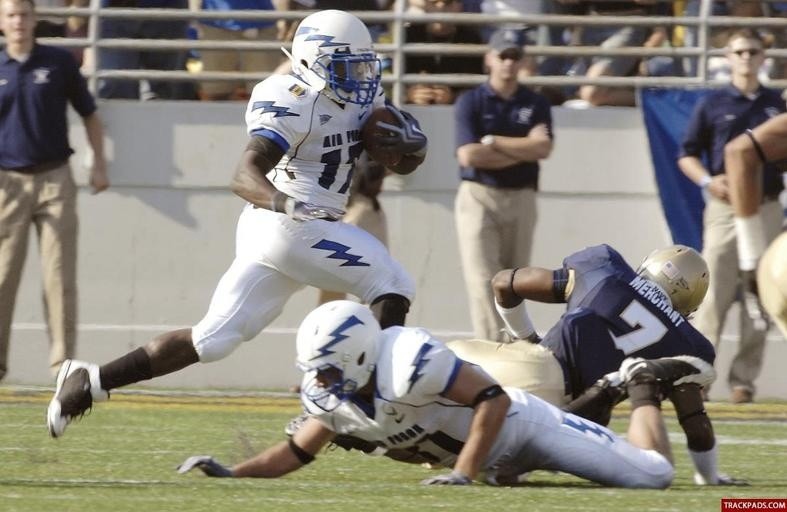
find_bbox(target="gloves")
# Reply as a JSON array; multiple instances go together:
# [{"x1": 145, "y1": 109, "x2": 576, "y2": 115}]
[
  {"x1": 421, "y1": 472, "x2": 472, "y2": 485},
  {"x1": 177, "y1": 456, "x2": 232, "y2": 477},
  {"x1": 370, "y1": 103, "x2": 427, "y2": 156},
  {"x1": 284, "y1": 198, "x2": 345, "y2": 221}
]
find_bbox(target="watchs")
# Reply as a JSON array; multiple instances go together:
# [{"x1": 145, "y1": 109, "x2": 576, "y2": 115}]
[{"x1": 481, "y1": 134, "x2": 495, "y2": 149}]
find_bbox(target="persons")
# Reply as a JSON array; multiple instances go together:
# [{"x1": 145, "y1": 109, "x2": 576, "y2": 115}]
[
  {"x1": 0, "y1": 0, "x2": 785, "y2": 101},
  {"x1": 173, "y1": 299, "x2": 718, "y2": 490},
  {"x1": 0, "y1": 0, "x2": 110, "y2": 385},
  {"x1": 673, "y1": 29, "x2": 785, "y2": 404},
  {"x1": 452, "y1": 28, "x2": 554, "y2": 343},
  {"x1": 47, "y1": 10, "x2": 429, "y2": 439},
  {"x1": 722, "y1": 114, "x2": 785, "y2": 339},
  {"x1": 445, "y1": 243, "x2": 747, "y2": 486}
]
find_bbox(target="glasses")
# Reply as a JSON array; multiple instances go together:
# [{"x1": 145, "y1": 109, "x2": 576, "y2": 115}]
[
  {"x1": 499, "y1": 52, "x2": 520, "y2": 59},
  {"x1": 736, "y1": 48, "x2": 758, "y2": 54}
]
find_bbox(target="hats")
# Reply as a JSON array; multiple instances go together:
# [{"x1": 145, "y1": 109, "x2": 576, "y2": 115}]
[{"x1": 488, "y1": 29, "x2": 525, "y2": 50}]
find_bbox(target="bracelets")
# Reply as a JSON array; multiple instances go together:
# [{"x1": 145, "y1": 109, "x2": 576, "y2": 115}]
[
  {"x1": 700, "y1": 174, "x2": 711, "y2": 189},
  {"x1": 734, "y1": 218, "x2": 763, "y2": 266},
  {"x1": 270, "y1": 190, "x2": 281, "y2": 213}
]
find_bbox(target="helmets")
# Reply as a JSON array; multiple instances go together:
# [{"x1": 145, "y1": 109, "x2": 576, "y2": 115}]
[
  {"x1": 296, "y1": 300, "x2": 382, "y2": 413},
  {"x1": 636, "y1": 244, "x2": 709, "y2": 316},
  {"x1": 280, "y1": 10, "x2": 382, "y2": 104}
]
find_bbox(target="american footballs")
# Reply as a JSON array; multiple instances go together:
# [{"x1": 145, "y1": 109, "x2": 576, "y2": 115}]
[{"x1": 362, "y1": 108, "x2": 412, "y2": 170}]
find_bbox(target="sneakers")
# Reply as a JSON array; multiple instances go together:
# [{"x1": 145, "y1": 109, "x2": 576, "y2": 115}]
[
  {"x1": 619, "y1": 354, "x2": 717, "y2": 388},
  {"x1": 47, "y1": 358, "x2": 110, "y2": 438}
]
